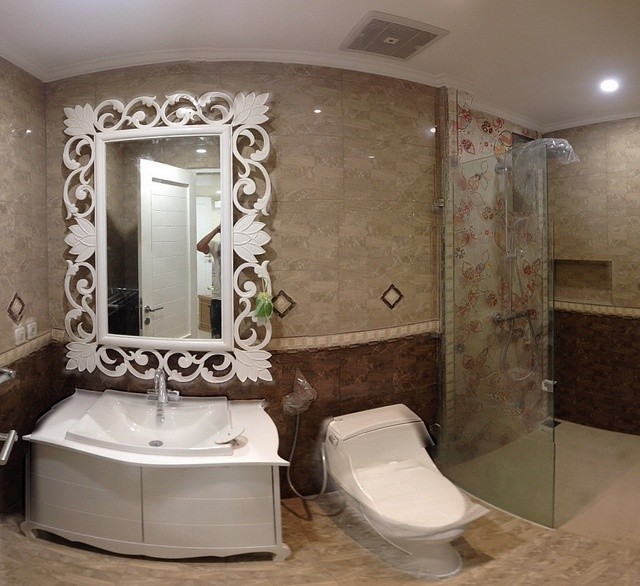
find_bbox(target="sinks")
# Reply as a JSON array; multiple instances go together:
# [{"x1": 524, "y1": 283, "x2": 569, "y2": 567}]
[{"x1": 66, "y1": 389, "x2": 233, "y2": 455}]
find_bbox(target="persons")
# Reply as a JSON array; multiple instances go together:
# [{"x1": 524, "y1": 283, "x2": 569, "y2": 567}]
[{"x1": 196, "y1": 223, "x2": 221, "y2": 339}]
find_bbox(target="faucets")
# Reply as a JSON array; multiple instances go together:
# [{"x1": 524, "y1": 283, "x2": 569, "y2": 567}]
[{"x1": 157, "y1": 371, "x2": 168, "y2": 403}]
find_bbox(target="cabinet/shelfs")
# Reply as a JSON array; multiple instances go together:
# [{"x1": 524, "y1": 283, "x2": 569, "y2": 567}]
[
  {"x1": 109, "y1": 287, "x2": 140, "y2": 315},
  {"x1": 142, "y1": 460, "x2": 273, "y2": 559},
  {"x1": 29, "y1": 441, "x2": 143, "y2": 556}
]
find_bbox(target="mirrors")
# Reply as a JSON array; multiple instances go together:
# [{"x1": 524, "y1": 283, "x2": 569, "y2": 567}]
[{"x1": 93, "y1": 124, "x2": 234, "y2": 352}]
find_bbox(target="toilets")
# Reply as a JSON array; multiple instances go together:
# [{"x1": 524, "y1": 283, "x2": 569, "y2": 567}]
[{"x1": 324, "y1": 404, "x2": 472, "y2": 577}]
